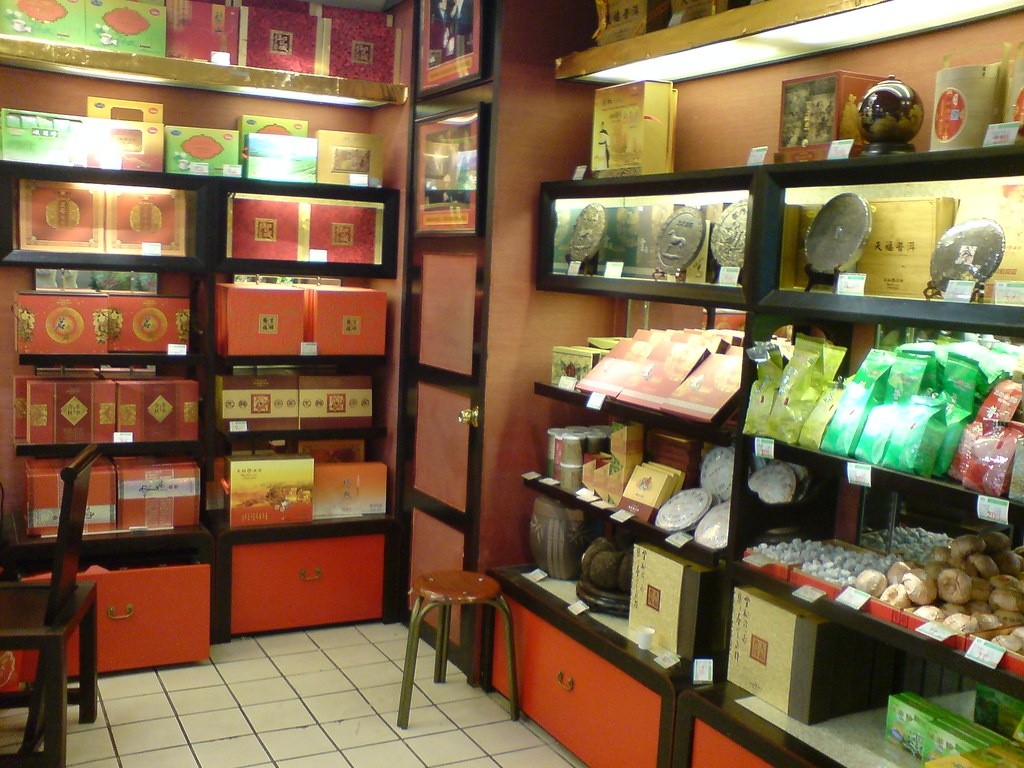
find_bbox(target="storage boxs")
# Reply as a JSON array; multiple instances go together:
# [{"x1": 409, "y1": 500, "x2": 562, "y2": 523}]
[
  {"x1": 667, "y1": 220, "x2": 717, "y2": 284},
  {"x1": 693, "y1": 202, "x2": 734, "y2": 284},
  {"x1": 590, "y1": 79, "x2": 673, "y2": 176},
  {"x1": 0, "y1": 0, "x2": 403, "y2": 536},
  {"x1": 596, "y1": 202, "x2": 687, "y2": 280},
  {"x1": 855, "y1": 195, "x2": 956, "y2": 296},
  {"x1": 793, "y1": 203, "x2": 826, "y2": 289},
  {"x1": 670, "y1": 0, "x2": 751, "y2": 25},
  {"x1": 778, "y1": 69, "x2": 887, "y2": 163},
  {"x1": 550, "y1": 327, "x2": 1024, "y2": 768},
  {"x1": 595, "y1": 0, "x2": 671, "y2": 47}
]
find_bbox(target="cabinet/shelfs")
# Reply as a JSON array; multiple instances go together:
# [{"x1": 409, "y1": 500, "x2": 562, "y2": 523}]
[
  {"x1": 477, "y1": 0, "x2": 1024, "y2": 768},
  {"x1": 0, "y1": 34, "x2": 408, "y2": 683}
]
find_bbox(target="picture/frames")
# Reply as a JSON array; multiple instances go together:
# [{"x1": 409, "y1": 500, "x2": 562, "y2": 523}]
[
  {"x1": 413, "y1": 0, "x2": 490, "y2": 100},
  {"x1": 411, "y1": 101, "x2": 486, "y2": 239}
]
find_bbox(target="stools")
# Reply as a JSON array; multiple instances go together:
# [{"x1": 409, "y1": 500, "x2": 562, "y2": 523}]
[{"x1": 397, "y1": 570, "x2": 521, "y2": 729}]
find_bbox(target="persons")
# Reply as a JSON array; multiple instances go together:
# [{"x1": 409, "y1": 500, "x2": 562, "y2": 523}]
[
  {"x1": 429, "y1": 0, "x2": 472, "y2": 63},
  {"x1": 424, "y1": 130, "x2": 469, "y2": 205}
]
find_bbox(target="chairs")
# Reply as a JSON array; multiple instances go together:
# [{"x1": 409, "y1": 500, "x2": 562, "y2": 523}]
[{"x1": 0, "y1": 443, "x2": 99, "y2": 768}]
